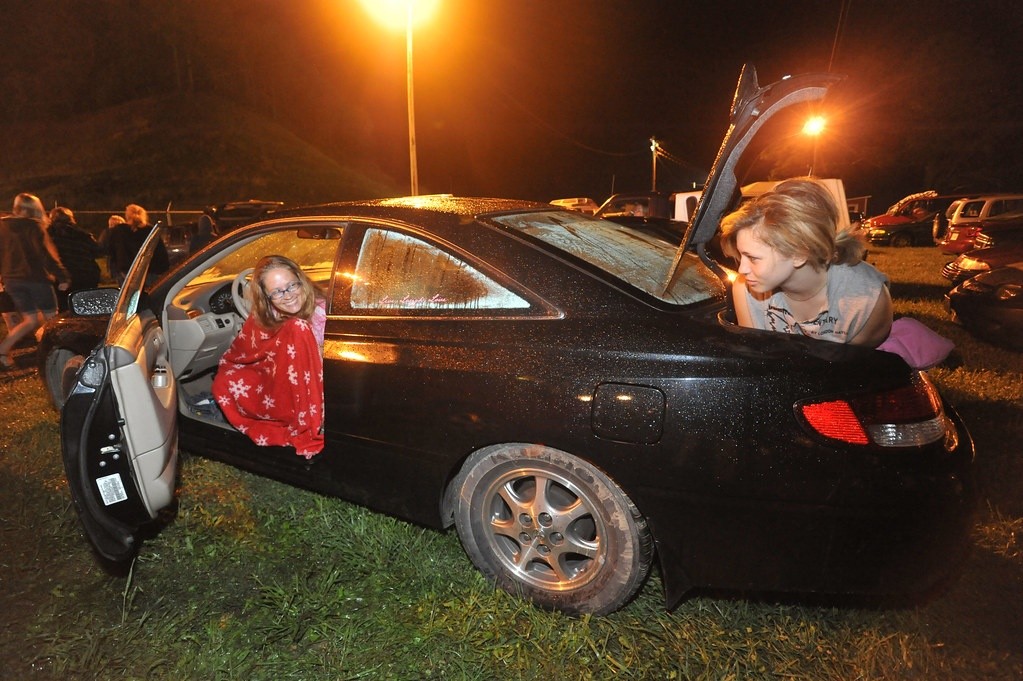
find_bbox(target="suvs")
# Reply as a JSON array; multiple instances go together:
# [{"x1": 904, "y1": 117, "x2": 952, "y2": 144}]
[{"x1": 932, "y1": 193, "x2": 1023, "y2": 254}]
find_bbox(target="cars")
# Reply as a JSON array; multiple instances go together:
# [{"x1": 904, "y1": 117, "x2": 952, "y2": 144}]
[
  {"x1": 941, "y1": 243, "x2": 1023, "y2": 286},
  {"x1": 35, "y1": 62, "x2": 977, "y2": 618},
  {"x1": 181, "y1": 199, "x2": 288, "y2": 242},
  {"x1": 944, "y1": 261, "x2": 1023, "y2": 345},
  {"x1": 593, "y1": 193, "x2": 670, "y2": 218},
  {"x1": 974, "y1": 205, "x2": 1023, "y2": 248},
  {"x1": 860, "y1": 194, "x2": 981, "y2": 247},
  {"x1": 549, "y1": 198, "x2": 606, "y2": 218}
]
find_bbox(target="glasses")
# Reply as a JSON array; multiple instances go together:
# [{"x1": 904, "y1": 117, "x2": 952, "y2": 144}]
[{"x1": 267, "y1": 281, "x2": 302, "y2": 300}]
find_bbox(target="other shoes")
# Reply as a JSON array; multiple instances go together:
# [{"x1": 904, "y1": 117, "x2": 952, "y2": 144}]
[{"x1": 0, "y1": 353, "x2": 18, "y2": 370}]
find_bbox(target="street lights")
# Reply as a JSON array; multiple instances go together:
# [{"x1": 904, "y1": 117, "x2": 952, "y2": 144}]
[{"x1": 801, "y1": 115, "x2": 827, "y2": 176}]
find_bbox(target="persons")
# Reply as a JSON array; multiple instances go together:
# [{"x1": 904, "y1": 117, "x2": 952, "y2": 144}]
[
  {"x1": 197, "y1": 201, "x2": 220, "y2": 245},
  {"x1": 45, "y1": 207, "x2": 104, "y2": 313},
  {"x1": 110, "y1": 204, "x2": 170, "y2": 289},
  {"x1": 213, "y1": 255, "x2": 328, "y2": 458},
  {"x1": 0, "y1": 193, "x2": 71, "y2": 366},
  {"x1": 719, "y1": 176, "x2": 894, "y2": 349},
  {"x1": 99, "y1": 215, "x2": 127, "y2": 288}
]
